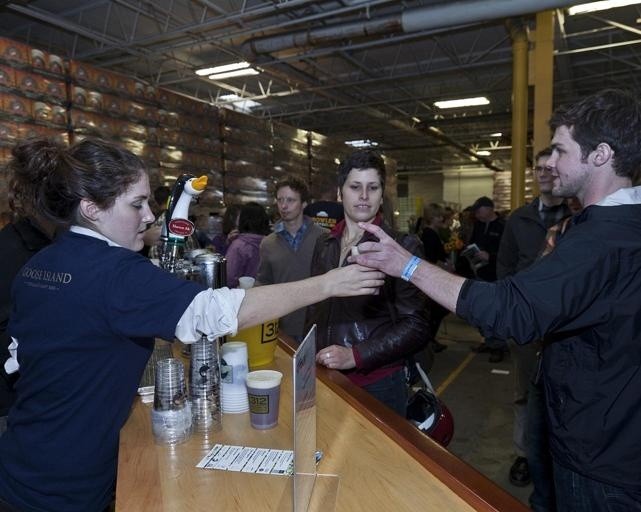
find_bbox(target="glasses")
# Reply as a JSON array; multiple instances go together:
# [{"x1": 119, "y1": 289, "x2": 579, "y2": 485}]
[{"x1": 532, "y1": 166, "x2": 553, "y2": 174}]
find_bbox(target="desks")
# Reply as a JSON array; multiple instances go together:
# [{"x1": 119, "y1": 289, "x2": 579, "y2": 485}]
[{"x1": 115, "y1": 330, "x2": 534, "y2": 511}]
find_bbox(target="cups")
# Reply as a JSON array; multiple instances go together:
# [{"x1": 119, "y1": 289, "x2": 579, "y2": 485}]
[
  {"x1": 142, "y1": 324, "x2": 281, "y2": 447},
  {"x1": 237, "y1": 276, "x2": 256, "y2": 288}
]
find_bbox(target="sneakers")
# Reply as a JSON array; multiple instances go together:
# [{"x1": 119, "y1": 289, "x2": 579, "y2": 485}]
[{"x1": 431, "y1": 339, "x2": 448, "y2": 354}]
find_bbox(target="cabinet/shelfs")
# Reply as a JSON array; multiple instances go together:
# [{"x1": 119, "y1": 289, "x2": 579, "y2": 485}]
[{"x1": 493, "y1": 169, "x2": 534, "y2": 213}]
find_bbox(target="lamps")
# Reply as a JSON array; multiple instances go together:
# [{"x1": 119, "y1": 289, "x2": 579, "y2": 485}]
[
  {"x1": 433, "y1": 96, "x2": 490, "y2": 109},
  {"x1": 568, "y1": 0, "x2": 641, "y2": 17}
]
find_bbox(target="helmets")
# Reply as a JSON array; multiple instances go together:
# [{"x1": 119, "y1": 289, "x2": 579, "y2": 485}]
[{"x1": 407, "y1": 391, "x2": 455, "y2": 448}]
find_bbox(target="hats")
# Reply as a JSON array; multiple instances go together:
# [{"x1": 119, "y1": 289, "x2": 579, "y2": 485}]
[{"x1": 471, "y1": 196, "x2": 495, "y2": 212}]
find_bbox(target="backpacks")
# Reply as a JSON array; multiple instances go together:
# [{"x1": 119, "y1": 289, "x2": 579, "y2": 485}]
[{"x1": 320, "y1": 314, "x2": 420, "y2": 387}]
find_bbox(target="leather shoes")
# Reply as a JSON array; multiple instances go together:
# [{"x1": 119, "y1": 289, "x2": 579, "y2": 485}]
[
  {"x1": 470, "y1": 343, "x2": 493, "y2": 354},
  {"x1": 510, "y1": 457, "x2": 532, "y2": 487},
  {"x1": 489, "y1": 349, "x2": 505, "y2": 362}
]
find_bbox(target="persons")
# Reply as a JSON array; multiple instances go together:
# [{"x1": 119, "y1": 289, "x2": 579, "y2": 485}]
[{"x1": 1, "y1": 89, "x2": 641, "y2": 511}]
